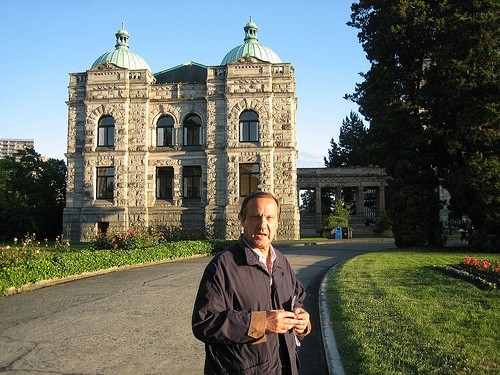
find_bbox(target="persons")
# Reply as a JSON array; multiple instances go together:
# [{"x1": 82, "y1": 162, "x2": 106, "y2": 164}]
[{"x1": 191, "y1": 192, "x2": 312, "y2": 375}]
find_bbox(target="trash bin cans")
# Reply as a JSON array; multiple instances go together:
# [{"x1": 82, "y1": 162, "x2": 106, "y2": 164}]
[
  {"x1": 335, "y1": 227, "x2": 342, "y2": 240},
  {"x1": 350, "y1": 228, "x2": 354, "y2": 239},
  {"x1": 342, "y1": 227, "x2": 350, "y2": 239}
]
